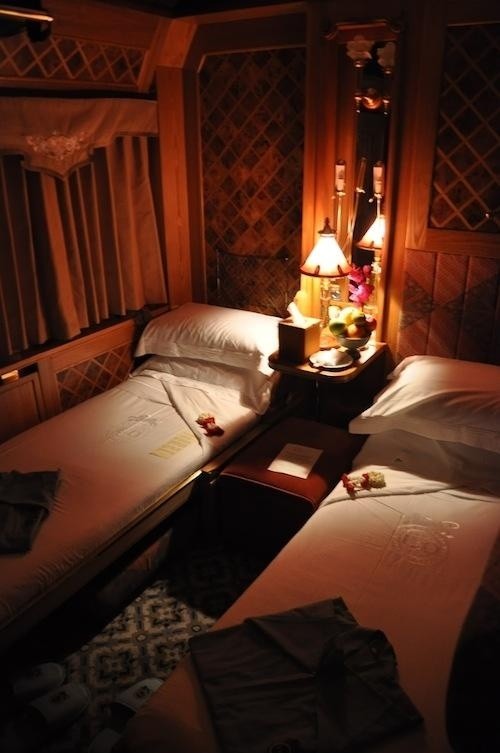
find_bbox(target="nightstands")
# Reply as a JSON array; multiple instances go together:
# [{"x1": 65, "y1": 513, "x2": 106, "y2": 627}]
[{"x1": 269, "y1": 339, "x2": 387, "y2": 424}]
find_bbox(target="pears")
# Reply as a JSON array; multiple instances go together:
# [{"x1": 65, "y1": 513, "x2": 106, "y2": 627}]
[
  {"x1": 351, "y1": 311, "x2": 366, "y2": 326},
  {"x1": 328, "y1": 316, "x2": 347, "y2": 337}
]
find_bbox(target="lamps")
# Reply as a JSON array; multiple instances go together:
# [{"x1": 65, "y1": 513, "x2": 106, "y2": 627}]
[
  {"x1": 355, "y1": 214, "x2": 388, "y2": 273},
  {"x1": 297, "y1": 220, "x2": 352, "y2": 329}
]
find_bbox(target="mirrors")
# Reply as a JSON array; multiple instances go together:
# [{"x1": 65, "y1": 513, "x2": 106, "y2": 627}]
[{"x1": 314, "y1": 21, "x2": 399, "y2": 342}]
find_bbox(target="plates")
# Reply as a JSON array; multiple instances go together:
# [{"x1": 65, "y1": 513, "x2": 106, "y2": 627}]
[{"x1": 309, "y1": 349, "x2": 354, "y2": 371}]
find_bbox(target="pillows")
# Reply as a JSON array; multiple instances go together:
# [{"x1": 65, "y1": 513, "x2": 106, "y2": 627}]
[
  {"x1": 342, "y1": 353, "x2": 498, "y2": 492},
  {"x1": 127, "y1": 296, "x2": 286, "y2": 415}
]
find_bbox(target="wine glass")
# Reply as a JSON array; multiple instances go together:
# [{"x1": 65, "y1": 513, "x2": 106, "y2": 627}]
[{"x1": 335, "y1": 333, "x2": 371, "y2": 360}]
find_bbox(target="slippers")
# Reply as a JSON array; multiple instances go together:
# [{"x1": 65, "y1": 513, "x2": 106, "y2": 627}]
[
  {"x1": 94, "y1": 676, "x2": 163, "y2": 748},
  {"x1": 11, "y1": 663, "x2": 89, "y2": 741}
]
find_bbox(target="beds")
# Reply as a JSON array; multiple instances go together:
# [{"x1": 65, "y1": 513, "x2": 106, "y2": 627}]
[
  {"x1": 117, "y1": 412, "x2": 500, "y2": 752},
  {"x1": 0, "y1": 305, "x2": 267, "y2": 642}
]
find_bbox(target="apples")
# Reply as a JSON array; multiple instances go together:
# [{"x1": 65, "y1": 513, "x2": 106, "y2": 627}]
[
  {"x1": 339, "y1": 307, "x2": 358, "y2": 326},
  {"x1": 345, "y1": 323, "x2": 366, "y2": 338},
  {"x1": 364, "y1": 314, "x2": 377, "y2": 332}
]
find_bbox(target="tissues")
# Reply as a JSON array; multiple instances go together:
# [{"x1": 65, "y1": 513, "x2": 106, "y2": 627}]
[{"x1": 278, "y1": 301, "x2": 322, "y2": 364}]
